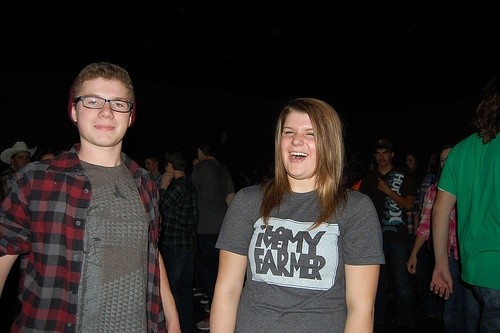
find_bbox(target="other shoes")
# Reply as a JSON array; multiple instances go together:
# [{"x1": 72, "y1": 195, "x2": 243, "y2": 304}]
[{"x1": 197, "y1": 317, "x2": 211, "y2": 330}]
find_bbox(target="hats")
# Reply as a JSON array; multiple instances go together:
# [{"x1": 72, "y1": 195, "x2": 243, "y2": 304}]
[
  {"x1": 378, "y1": 139, "x2": 393, "y2": 151},
  {"x1": 0, "y1": 141, "x2": 37, "y2": 164}
]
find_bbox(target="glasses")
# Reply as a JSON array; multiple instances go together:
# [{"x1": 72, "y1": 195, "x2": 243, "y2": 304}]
[{"x1": 74, "y1": 94, "x2": 134, "y2": 114}]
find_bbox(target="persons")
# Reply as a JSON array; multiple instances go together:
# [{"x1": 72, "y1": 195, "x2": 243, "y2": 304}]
[
  {"x1": 430, "y1": 78, "x2": 500, "y2": 333},
  {"x1": 0, "y1": 62, "x2": 181, "y2": 333},
  {"x1": 0, "y1": 141, "x2": 37, "y2": 203},
  {"x1": 37, "y1": 149, "x2": 58, "y2": 160},
  {"x1": 339, "y1": 137, "x2": 461, "y2": 333},
  {"x1": 156, "y1": 149, "x2": 199, "y2": 333},
  {"x1": 207, "y1": 97, "x2": 387, "y2": 333},
  {"x1": 190, "y1": 141, "x2": 235, "y2": 331},
  {"x1": 145, "y1": 154, "x2": 163, "y2": 184}
]
